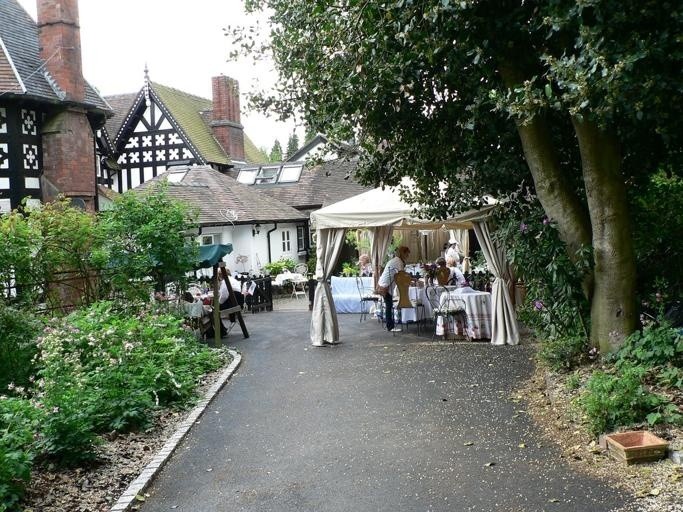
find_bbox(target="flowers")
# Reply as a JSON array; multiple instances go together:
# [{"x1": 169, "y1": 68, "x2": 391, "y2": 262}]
[{"x1": 423, "y1": 261, "x2": 435, "y2": 276}]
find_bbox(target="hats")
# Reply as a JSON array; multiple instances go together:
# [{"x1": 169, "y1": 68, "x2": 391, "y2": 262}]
[{"x1": 449, "y1": 238, "x2": 458, "y2": 244}]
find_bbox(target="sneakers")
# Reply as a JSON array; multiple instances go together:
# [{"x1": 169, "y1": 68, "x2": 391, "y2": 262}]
[{"x1": 391, "y1": 327, "x2": 402, "y2": 333}]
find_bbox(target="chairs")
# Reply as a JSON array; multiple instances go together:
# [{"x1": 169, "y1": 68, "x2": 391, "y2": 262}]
[
  {"x1": 293, "y1": 263, "x2": 309, "y2": 279},
  {"x1": 355, "y1": 272, "x2": 385, "y2": 323},
  {"x1": 424, "y1": 286, "x2": 470, "y2": 343},
  {"x1": 393, "y1": 270, "x2": 425, "y2": 338},
  {"x1": 182, "y1": 281, "x2": 202, "y2": 292}
]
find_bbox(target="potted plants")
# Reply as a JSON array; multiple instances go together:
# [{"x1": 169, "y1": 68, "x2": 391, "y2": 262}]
[{"x1": 342, "y1": 262, "x2": 353, "y2": 277}]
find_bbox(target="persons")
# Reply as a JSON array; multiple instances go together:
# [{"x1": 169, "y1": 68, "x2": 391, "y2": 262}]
[
  {"x1": 377, "y1": 247, "x2": 411, "y2": 332},
  {"x1": 446, "y1": 257, "x2": 457, "y2": 267},
  {"x1": 140, "y1": 269, "x2": 256, "y2": 337},
  {"x1": 358, "y1": 254, "x2": 373, "y2": 275},
  {"x1": 444, "y1": 238, "x2": 460, "y2": 267},
  {"x1": 437, "y1": 258, "x2": 466, "y2": 282}
]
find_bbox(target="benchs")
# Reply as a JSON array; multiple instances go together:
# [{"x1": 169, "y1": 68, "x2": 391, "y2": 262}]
[{"x1": 331, "y1": 276, "x2": 374, "y2": 313}]
